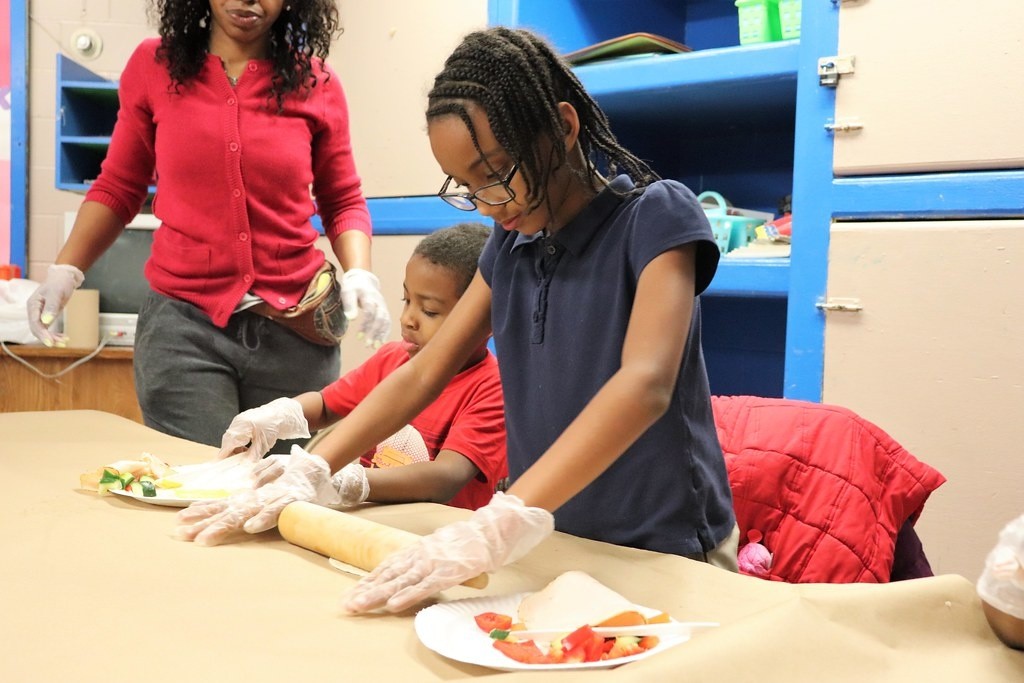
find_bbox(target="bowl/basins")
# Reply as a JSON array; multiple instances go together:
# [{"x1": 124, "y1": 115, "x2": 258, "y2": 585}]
[{"x1": 981, "y1": 596, "x2": 1024, "y2": 651}]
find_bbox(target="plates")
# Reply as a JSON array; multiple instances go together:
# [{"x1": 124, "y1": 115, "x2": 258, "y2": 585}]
[
  {"x1": 414, "y1": 593, "x2": 690, "y2": 668},
  {"x1": 110, "y1": 464, "x2": 246, "y2": 507}
]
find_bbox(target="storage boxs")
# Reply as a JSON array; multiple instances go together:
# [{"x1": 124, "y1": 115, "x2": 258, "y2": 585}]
[{"x1": 733, "y1": 0, "x2": 801, "y2": 45}]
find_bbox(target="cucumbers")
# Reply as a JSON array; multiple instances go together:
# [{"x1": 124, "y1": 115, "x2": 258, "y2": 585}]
[
  {"x1": 122, "y1": 473, "x2": 135, "y2": 485},
  {"x1": 140, "y1": 476, "x2": 156, "y2": 486},
  {"x1": 100, "y1": 479, "x2": 122, "y2": 489},
  {"x1": 132, "y1": 482, "x2": 156, "y2": 497}
]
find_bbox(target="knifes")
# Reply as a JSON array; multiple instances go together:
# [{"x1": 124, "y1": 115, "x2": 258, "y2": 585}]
[{"x1": 511, "y1": 623, "x2": 719, "y2": 642}]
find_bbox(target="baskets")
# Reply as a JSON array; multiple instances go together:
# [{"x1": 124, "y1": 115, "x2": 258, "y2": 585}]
[{"x1": 695, "y1": 191, "x2": 768, "y2": 257}]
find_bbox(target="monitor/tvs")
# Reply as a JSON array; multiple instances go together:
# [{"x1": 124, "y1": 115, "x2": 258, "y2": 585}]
[{"x1": 64, "y1": 212, "x2": 166, "y2": 346}]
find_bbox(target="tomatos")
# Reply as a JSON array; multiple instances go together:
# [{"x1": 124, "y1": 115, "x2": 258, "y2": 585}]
[
  {"x1": 562, "y1": 626, "x2": 593, "y2": 650},
  {"x1": 475, "y1": 612, "x2": 512, "y2": 632},
  {"x1": 585, "y1": 638, "x2": 604, "y2": 661},
  {"x1": 494, "y1": 642, "x2": 550, "y2": 664},
  {"x1": 604, "y1": 637, "x2": 650, "y2": 655}
]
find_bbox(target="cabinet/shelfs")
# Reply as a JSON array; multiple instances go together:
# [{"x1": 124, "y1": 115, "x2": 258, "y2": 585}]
[{"x1": 569, "y1": 38, "x2": 802, "y2": 296}]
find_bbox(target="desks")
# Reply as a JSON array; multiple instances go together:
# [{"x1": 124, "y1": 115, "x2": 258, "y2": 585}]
[
  {"x1": 0, "y1": 344, "x2": 146, "y2": 424},
  {"x1": 0, "y1": 408, "x2": 1024, "y2": 683}
]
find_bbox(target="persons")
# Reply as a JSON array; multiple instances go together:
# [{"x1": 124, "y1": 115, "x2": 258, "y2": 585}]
[
  {"x1": 28, "y1": 0, "x2": 392, "y2": 459},
  {"x1": 215, "y1": 227, "x2": 513, "y2": 510},
  {"x1": 177, "y1": 25, "x2": 744, "y2": 615}
]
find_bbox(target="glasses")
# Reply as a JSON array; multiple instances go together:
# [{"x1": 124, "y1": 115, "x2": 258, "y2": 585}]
[{"x1": 437, "y1": 155, "x2": 526, "y2": 212}]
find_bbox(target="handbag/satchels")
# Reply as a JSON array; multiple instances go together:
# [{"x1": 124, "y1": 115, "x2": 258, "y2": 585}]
[{"x1": 274, "y1": 258, "x2": 350, "y2": 348}]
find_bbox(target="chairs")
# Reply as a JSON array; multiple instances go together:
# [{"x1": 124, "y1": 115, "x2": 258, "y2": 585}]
[{"x1": 709, "y1": 394, "x2": 946, "y2": 583}]
